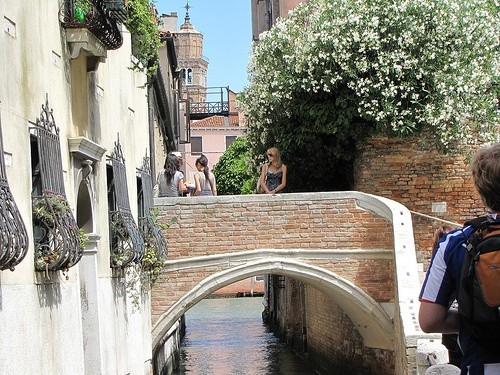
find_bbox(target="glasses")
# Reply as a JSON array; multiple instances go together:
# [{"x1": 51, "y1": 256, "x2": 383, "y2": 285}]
[{"x1": 268, "y1": 153, "x2": 273, "y2": 156}]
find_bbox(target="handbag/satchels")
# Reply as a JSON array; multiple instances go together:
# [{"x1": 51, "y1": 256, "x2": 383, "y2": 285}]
[
  {"x1": 256, "y1": 164, "x2": 268, "y2": 193},
  {"x1": 152, "y1": 169, "x2": 163, "y2": 197}
]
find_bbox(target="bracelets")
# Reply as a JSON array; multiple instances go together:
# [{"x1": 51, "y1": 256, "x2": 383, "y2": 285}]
[{"x1": 274, "y1": 189, "x2": 277, "y2": 192}]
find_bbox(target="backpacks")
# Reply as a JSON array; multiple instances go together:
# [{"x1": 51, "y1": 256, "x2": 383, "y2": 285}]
[{"x1": 457, "y1": 216, "x2": 499, "y2": 364}]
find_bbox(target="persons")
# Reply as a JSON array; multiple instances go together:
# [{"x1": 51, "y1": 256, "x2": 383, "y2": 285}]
[
  {"x1": 418, "y1": 142, "x2": 500, "y2": 375},
  {"x1": 190, "y1": 156, "x2": 217, "y2": 196},
  {"x1": 260, "y1": 147, "x2": 286, "y2": 194},
  {"x1": 157, "y1": 151, "x2": 192, "y2": 197}
]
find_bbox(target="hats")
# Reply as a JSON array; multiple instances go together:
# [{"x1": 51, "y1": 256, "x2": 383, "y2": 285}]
[{"x1": 171, "y1": 152, "x2": 182, "y2": 156}]
